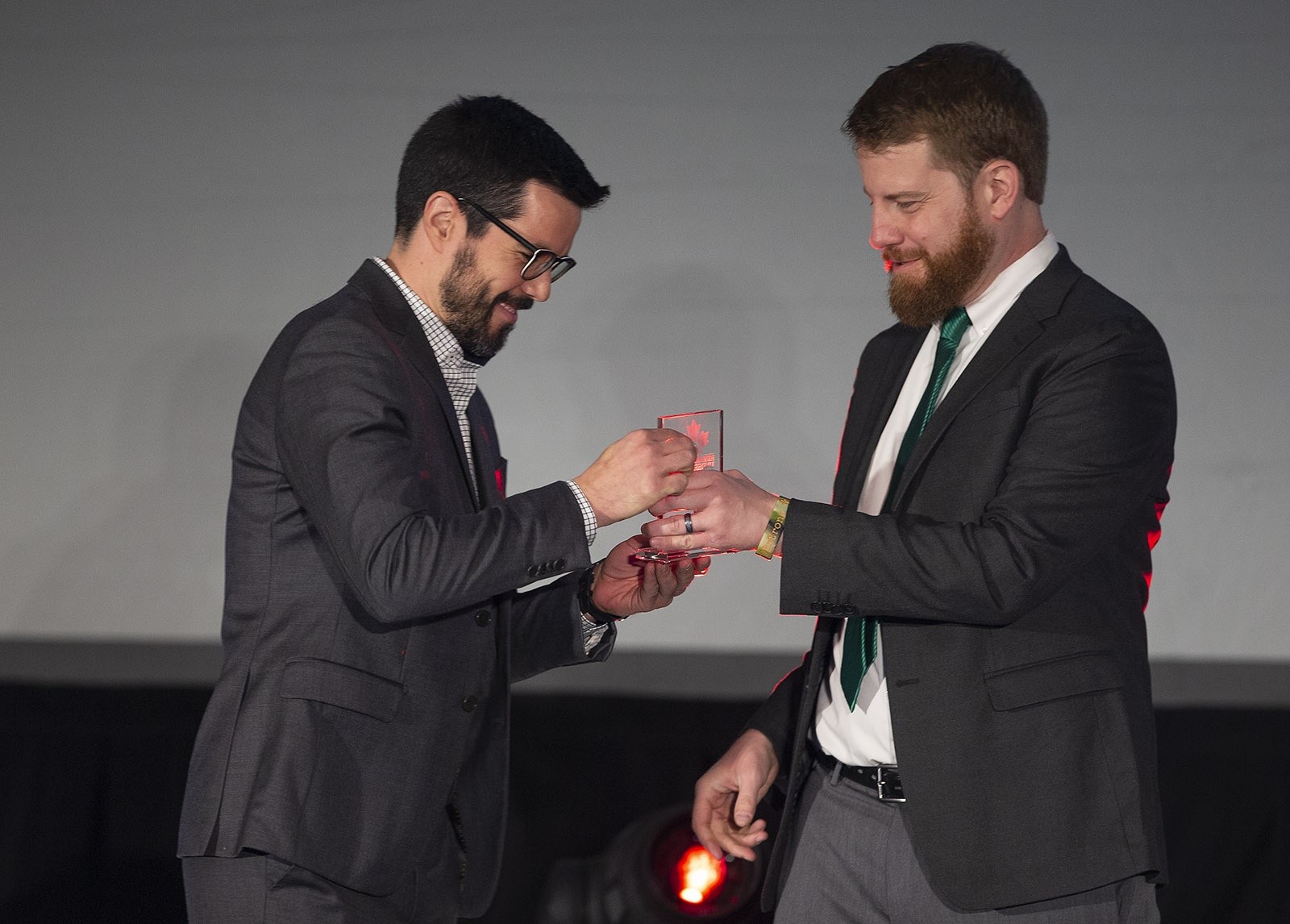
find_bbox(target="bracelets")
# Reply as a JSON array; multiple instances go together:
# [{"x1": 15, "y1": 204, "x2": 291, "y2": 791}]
[
  {"x1": 757, "y1": 495, "x2": 790, "y2": 559},
  {"x1": 581, "y1": 562, "x2": 630, "y2": 623}
]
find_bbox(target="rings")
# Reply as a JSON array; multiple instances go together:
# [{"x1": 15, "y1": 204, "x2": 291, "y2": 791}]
[{"x1": 684, "y1": 513, "x2": 693, "y2": 533}]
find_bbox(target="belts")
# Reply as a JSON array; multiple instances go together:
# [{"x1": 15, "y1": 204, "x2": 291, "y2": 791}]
[{"x1": 812, "y1": 746, "x2": 906, "y2": 802}]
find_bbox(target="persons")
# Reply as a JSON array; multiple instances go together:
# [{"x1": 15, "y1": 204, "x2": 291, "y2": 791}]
[
  {"x1": 176, "y1": 95, "x2": 710, "y2": 924},
  {"x1": 638, "y1": 43, "x2": 1178, "y2": 923}
]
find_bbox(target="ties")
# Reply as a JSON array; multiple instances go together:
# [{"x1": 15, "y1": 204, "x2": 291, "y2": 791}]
[{"x1": 840, "y1": 305, "x2": 972, "y2": 712}]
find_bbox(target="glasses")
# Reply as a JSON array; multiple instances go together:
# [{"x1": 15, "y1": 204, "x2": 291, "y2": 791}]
[{"x1": 455, "y1": 196, "x2": 576, "y2": 285}]
